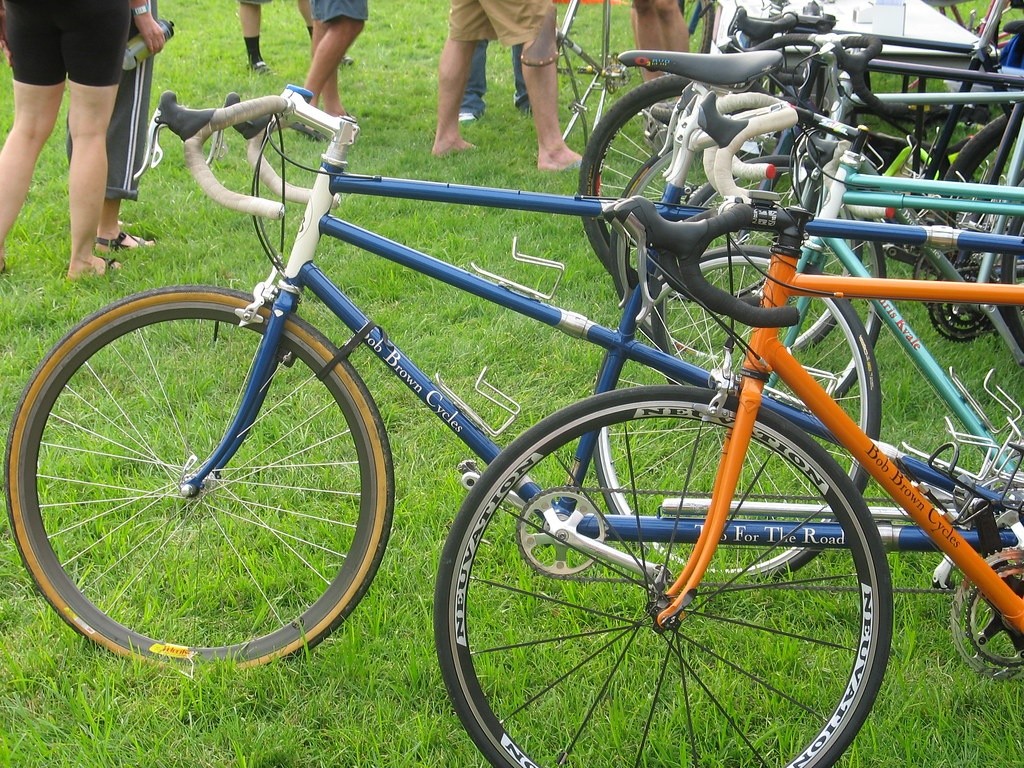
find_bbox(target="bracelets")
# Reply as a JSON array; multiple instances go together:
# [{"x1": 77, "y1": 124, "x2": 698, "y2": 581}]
[{"x1": 132, "y1": 5, "x2": 148, "y2": 15}]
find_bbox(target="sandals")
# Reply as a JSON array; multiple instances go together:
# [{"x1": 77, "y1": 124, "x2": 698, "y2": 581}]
[{"x1": 96, "y1": 233, "x2": 154, "y2": 251}]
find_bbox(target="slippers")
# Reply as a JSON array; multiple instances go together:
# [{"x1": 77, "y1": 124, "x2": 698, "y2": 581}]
[
  {"x1": 0, "y1": 257, "x2": 7, "y2": 276},
  {"x1": 99, "y1": 257, "x2": 119, "y2": 279}
]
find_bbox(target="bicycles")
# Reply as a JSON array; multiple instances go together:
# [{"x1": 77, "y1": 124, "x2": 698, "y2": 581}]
[{"x1": 7, "y1": 0, "x2": 1024, "y2": 767}]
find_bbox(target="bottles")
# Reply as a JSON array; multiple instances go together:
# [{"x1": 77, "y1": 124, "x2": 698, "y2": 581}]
[{"x1": 123, "y1": 18, "x2": 175, "y2": 70}]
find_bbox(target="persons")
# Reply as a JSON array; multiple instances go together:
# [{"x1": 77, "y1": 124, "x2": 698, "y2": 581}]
[
  {"x1": 0, "y1": 0, "x2": 122, "y2": 277},
  {"x1": 632, "y1": 0, "x2": 690, "y2": 138},
  {"x1": 458, "y1": 39, "x2": 532, "y2": 122},
  {"x1": 280, "y1": 0, "x2": 368, "y2": 140},
  {"x1": 239, "y1": 0, "x2": 354, "y2": 76},
  {"x1": 67, "y1": 0, "x2": 164, "y2": 255},
  {"x1": 433, "y1": 0, "x2": 583, "y2": 171}
]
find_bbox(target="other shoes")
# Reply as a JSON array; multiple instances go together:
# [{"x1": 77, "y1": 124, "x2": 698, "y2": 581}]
[
  {"x1": 246, "y1": 62, "x2": 277, "y2": 75},
  {"x1": 340, "y1": 55, "x2": 354, "y2": 66},
  {"x1": 458, "y1": 112, "x2": 476, "y2": 122}
]
